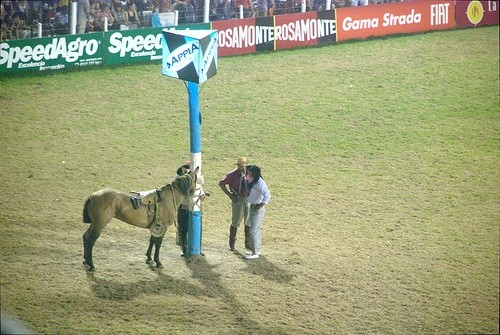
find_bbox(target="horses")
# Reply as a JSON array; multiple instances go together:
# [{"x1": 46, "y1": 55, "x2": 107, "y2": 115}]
[{"x1": 83, "y1": 165, "x2": 199, "y2": 272}]
